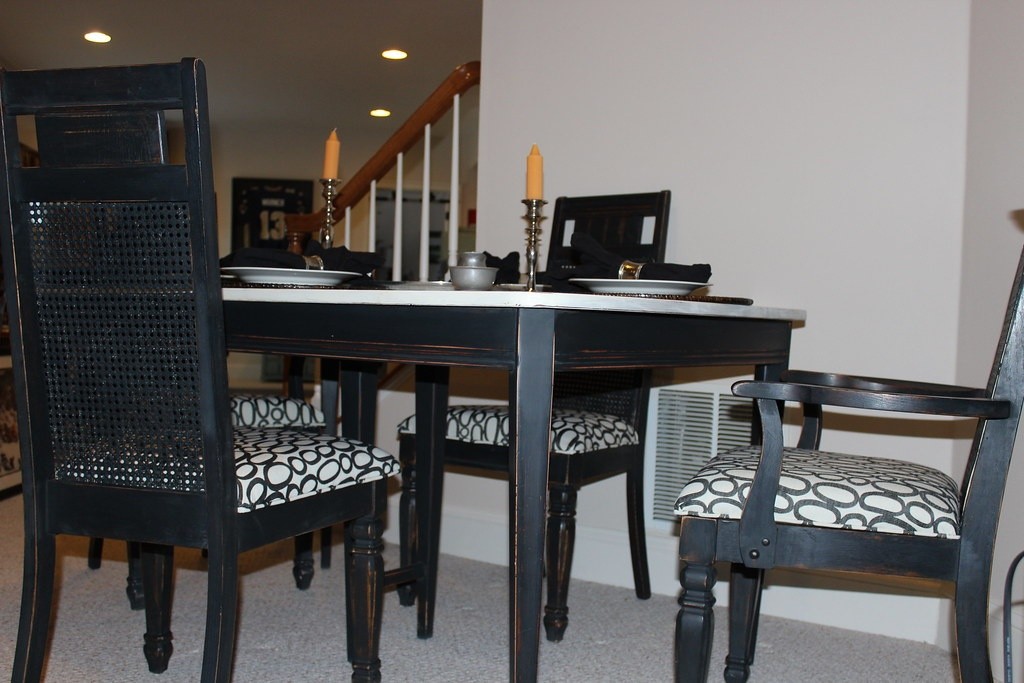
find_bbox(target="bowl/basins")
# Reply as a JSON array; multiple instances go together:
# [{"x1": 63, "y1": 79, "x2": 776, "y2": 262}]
[{"x1": 449, "y1": 265, "x2": 499, "y2": 290}]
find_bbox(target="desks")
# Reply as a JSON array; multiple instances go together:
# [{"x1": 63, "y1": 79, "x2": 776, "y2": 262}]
[{"x1": 216, "y1": 276, "x2": 796, "y2": 682}]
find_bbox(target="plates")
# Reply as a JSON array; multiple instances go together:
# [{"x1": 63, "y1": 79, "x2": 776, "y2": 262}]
[
  {"x1": 569, "y1": 279, "x2": 713, "y2": 295},
  {"x1": 219, "y1": 267, "x2": 363, "y2": 285},
  {"x1": 496, "y1": 284, "x2": 552, "y2": 292}
]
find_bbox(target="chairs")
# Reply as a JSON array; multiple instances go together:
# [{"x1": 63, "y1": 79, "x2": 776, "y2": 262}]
[
  {"x1": 3, "y1": 55, "x2": 401, "y2": 682},
  {"x1": 61, "y1": 108, "x2": 323, "y2": 591},
  {"x1": 394, "y1": 188, "x2": 673, "y2": 643},
  {"x1": 669, "y1": 236, "x2": 1024, "y2": 683}
]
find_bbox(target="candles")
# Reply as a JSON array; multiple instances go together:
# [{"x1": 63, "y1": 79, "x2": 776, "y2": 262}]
[
  {"x1": 323, "y1": 126, "x2": 339, "y2": 178},
  {"x1": 523, "y1": 144, "x2": 545, "y2": 200}
]
